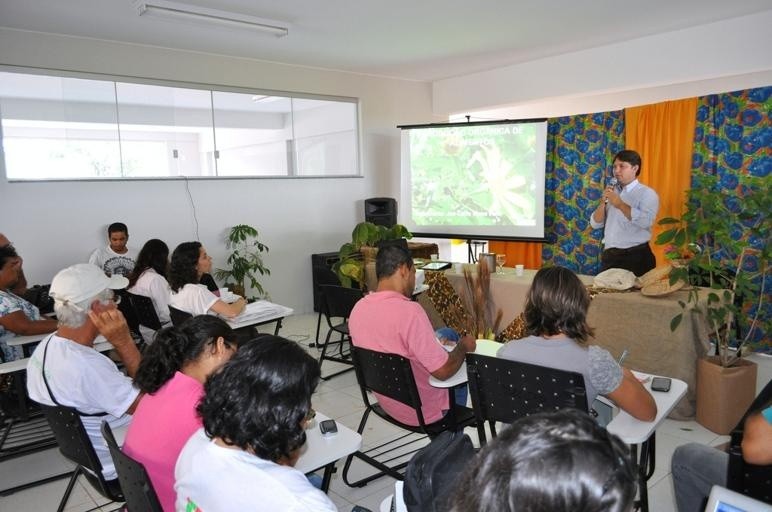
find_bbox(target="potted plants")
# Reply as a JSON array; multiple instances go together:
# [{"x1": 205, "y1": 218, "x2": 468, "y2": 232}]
[
  {"x1": 212, "y1": 225, "x2": 270, "y2": 298},
  {"x1": 655, "y1": 189, "x2": 770, "y2": 435}
]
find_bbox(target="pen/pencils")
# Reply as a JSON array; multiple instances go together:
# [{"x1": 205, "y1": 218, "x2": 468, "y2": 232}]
[{"x1": 618, "y1": 350, "x2": 627, "y2": 364}]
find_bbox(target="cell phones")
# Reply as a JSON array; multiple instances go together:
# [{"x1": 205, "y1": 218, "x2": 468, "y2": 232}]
[
  {"x1": 318, "y1": 419, "x2": 338, "y2": 436},
  {"x1": 651, "y1": 377, "x2": 673, "y2": 393}
]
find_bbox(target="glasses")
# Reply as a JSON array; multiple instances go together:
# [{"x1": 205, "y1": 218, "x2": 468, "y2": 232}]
[
  {"x1": 205, "y1": 338, "x2": 238, "y2": 355},
  {"x1": 107, "y1": 296, "x2": 122, "y2": 306}
]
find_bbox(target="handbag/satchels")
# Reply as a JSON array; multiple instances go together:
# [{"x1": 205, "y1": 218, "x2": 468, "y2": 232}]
[
  {"x1": 401, "y1": 429, "x2": 474, "y2": 512},
  {"x1": 39, "y1": 334, "x2": 107, "y2": 418}
]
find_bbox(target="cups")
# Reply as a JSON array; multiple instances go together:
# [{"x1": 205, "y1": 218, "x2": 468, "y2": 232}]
[
  {"x1": 430, "y1": 253, "x2": 437, "y2": 262},
  {"x1": 515, "y1": 264, "x2": 525, "y2": 277}
]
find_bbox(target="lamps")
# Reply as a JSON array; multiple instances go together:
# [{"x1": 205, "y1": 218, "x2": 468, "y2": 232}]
[{"x1": 133, "y1": 1, "x2": 288, "y2": 38}]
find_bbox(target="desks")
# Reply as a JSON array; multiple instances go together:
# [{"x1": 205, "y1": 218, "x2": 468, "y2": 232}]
[
  {"x1": 431, "y1": 330, "x2": 505, "y2": 388},
  {"x1": 225, "y1": 300, "x2": 295, "y2": 339},
  {"x1": 366, "y1": 259, "x2": 735, "y2": 420},
  {"x1": 301, "y1": 412, "x2": 360, "y2": 495},
  {"x1": 0, "y1": 329, "x2": 142, "y2": 463},
  {"x1": 587, "y1": 364, "x2": 690, "y2": 483}
]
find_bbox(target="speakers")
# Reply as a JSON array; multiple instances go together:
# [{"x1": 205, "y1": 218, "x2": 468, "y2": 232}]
[
  {"x1": 365, "y1": 197, "x2": 398, "y2": 228},
  {"x1": 313, "y1": 252, "x2": 339, "y2": 312}
]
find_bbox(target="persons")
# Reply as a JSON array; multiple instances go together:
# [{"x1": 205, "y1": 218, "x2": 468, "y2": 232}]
[
  {"x1": 200, "y1": 272, "x2": 221, "y2": 297},
  {"x1": 348, "y1": 246, "x2": 476, "y2": 442},
  {"x1": 0, "y1": 242, "x2": 59, "y2": 365},
  {"x1": 27, "y1": 263, "x2": 144, "y2": 498},
  {"x1": 496, "y1": 266, "x2": 658, "y2": 430},
  {"x1": 120, "y1": 314, "x2": 238, "y2": 512},
  {"x1": 432, "y1": 409, "x2": 636, "y2": 512},
  {"x1": 88, "y1": 222, "x2": 137, "y2": 278},
  {"x1": 167, "y1": 241, "x2": 259, "y2": 335},
  {"x1": 670, "y1": 378, "x2": 772, "y2": 512},
  {"x1": 589, "y1": 150, "x2": 659, "y2": 277},
  {"x1": 174, "y1": 333, "x2": 339, "y2": 512},
  {"x1": 125, "y1": 239, "x2": 174, "y2": 348}
]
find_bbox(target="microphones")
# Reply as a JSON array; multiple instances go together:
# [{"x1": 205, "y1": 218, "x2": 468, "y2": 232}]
[{"x1": 604, "y1": 177, "x2": 618, "y2": 204}]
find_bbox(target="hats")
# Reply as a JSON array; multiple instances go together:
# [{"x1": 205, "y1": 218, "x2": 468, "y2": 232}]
[{"x1": 48, "y1": 263, "x2": 129, "y2": 313}]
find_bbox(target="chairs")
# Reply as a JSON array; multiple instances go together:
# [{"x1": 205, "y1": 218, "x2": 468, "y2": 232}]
[
  {"x1": 166, "y1": 304, "x2": 194, "y2": 326},
  {"x1": 316, "y1": 283, "x2": 363, "y2": 380},
  {"x1": 463, "y1": 351, "x2": 656, "y2": 511},
  {"x1": 342, "y1": 346, "x2": 478, "y2": 489},
  {"x1": 99, "y1": 420, "x2": 162, "y2": 511},
  {"x1": 28, "y1": 402, "x2": 126, "y2": 512},
  {"x1": 127, "y1": 290, "x2": 165, "y2": 336}
]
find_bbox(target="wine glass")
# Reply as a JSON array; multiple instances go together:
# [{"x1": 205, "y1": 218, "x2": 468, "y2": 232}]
[{"x1": 495, "y1": 254, "x2": 509, "y2": 276}]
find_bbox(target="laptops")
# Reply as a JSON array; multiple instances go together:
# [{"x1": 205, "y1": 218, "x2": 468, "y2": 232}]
[{"x1": 703, "y1": 485, "x2": 772, "y2": 511}]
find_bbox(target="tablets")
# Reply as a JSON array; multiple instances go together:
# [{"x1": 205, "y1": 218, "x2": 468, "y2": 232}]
[{"x1": 419, "y1": 260, "x2": 452, "y2": 271}]
